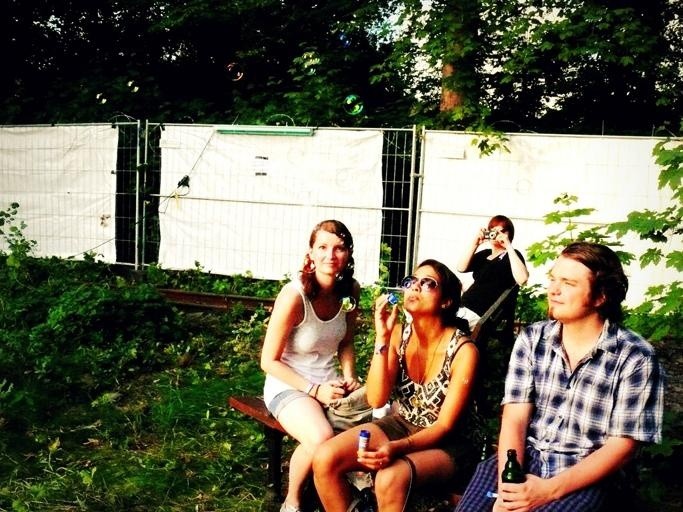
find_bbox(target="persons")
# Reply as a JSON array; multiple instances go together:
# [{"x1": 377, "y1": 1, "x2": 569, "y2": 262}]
[
  {"x1": 258, "y1": 219, "x2": 363, "y2": 512},
  {"x1": 454, "y1": 241, "x2": 665, "y2": 511},
  {"x1": 458, "y1": 215, "x2": 530, "y2": 347},
  {"x1": 308, "y1": 258, "x2": 481, "y2": 511}
]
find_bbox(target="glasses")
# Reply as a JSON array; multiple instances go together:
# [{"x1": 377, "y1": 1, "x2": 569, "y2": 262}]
[{"x1": 401, "y1": 274, "x2": 442, "y2": 294}]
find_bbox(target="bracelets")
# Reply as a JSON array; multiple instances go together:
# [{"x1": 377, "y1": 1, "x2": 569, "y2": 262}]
[
  {"x1": 371, "y1": 341, "x2": 390, "y2": 355},
  {"x1": 305, "y1": 383, "x2": 314, "y2": 394},
  {"x1": 402, "y1": 436, "x2": 416, "y2": 453},
  {"x1": 314, "y1": 384, "x2": 321, "y2": 398}
]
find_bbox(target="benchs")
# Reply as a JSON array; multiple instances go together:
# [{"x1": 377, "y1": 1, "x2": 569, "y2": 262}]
[
  {"x1": 229, "y1": 394, "x2": 370, "y2": 494},
  {"x1": 468, "y1": 280, "x2": 518, "y2": 394}
]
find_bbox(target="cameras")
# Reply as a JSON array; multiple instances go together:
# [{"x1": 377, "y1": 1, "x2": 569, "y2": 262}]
[{"x1": 484, "y1": 229, "x2": 499, "y2": 240}]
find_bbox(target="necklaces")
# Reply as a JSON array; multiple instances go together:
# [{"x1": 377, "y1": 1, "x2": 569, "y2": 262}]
[{"x1": 414, "y1": 326, "x2": 446, "y2": 383}]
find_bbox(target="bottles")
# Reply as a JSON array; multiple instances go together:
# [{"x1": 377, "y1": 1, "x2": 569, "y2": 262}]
[
  {"x1": 358, "y1": 428, "x2": 370, "y2": 451},
  {"x1": 500, "y1": 448, "x2": 525, "y2": 502}
]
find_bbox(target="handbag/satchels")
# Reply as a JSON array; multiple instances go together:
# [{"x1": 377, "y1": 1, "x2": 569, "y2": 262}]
[
  {"x1": 326, "y1": 384, "x2": 372, "y2": 431},
  {"x1": 457, "y1": 305, "x2": 483, "y2": 346}
]
